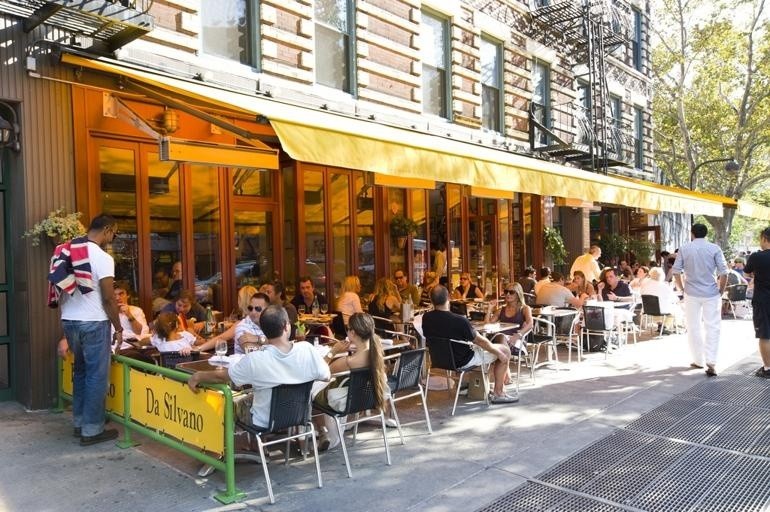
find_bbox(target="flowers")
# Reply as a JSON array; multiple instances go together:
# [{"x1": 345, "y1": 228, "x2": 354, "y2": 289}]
[
  {"x1": 19, "y1": 207, "x2": 90, "y2": 248},
  {"x1": 387, "y1": 215, "x2": 420, "y2": 237},
  {"x1": 544, "y1": 229, "x2": 569, "y2": 267}
]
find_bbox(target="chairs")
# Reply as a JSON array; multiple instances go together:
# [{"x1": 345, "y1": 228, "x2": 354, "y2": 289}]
[
  {"x1": 234, "y1": 378, "x2": 325, "y2": 506},
  {"x1": 308, "y1": 366, "x2": 395, "y2": 480}
]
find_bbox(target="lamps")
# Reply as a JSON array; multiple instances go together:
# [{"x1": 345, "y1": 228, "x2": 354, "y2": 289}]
[{"x1": 1, "y1": 101, "x2": 26, "y2": 156}]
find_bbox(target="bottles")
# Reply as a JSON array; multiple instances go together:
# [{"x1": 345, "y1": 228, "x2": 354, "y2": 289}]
[
  {"x1": 204, "y1": 305, "x2": 213, "y2": 334},
  {"x1": 313, "y1": 295, "x2": 319, "y2": 314},
  {"x1": 312, "y1": 337, "x2": 320, "y2": 349},
  {"x1": 408, "y1": 293, "x2": 415, "y2": 322}
]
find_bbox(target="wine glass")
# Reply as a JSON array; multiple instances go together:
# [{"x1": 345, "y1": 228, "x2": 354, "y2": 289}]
[
  {"x1": 320, "y1": 303, "x2": 328, "y2": 317},
  {"x1": 214, "y1": 339, "x2": 227, "y2": 369},
  {"x1": 298, "y1": 305, "x2": 305, "y2": 319}
]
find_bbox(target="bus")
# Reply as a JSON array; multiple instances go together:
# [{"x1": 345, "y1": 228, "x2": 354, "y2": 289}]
[{"x1": 105, "y1": 231, "x2": 260, "y2": 298}]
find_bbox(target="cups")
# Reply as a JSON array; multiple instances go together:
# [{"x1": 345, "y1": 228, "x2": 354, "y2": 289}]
[
  {"x1": 418, "y1": 304, "x2": 435, "y2": 315},
  {"x1": 295, "y1": 329, "x2": 304, "y2": 342},
  {"x1": 245, "y1": 345, "x2": 255, "y2": 353}
]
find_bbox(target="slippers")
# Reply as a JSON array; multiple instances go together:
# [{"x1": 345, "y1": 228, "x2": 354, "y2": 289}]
[
  {"x1": 691, "y1": 362, "x2": 703, "y2": 368},
  {"x1": 706, "y1": 368, "x2": 716, "y2": 376}
]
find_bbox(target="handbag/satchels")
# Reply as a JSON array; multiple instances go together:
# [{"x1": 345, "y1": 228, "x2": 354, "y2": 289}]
[
  {"x1": 399, "y1": 296, "x2": 415, "y2": 324},
  {"x1": 328, "y1": 378, "x2": 351, "y2": 413}
]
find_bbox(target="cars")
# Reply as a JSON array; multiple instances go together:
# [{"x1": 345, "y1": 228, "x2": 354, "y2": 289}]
[{"x1": 194, "y1": 259, "x2": 327, "y2": 311}]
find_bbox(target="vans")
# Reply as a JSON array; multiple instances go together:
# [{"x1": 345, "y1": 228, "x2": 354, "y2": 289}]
[{"x1": 357, "y1": 234, "x2": 457, "y2": 292}]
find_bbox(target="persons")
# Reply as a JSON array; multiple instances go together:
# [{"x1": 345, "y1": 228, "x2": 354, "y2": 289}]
[
  {"x1": 660, "y1": 251, "x2": 679, "y2": 269},
  {"x1": 671, "y1": 223, "x2": 729, "y2": 378},
  {"x1": 741, "y1": 227, "x2": 770, "y2": 379},
  {"x1": 732, "y1": 251, "x2": 751, "y2": 275},
  {"x1": 430, "y1": 244, "x2": 447, "y2": 285},
  {"x1": 569, "y1": 246, "x2": 602, "y2": 282},
  {"x1": 109, "y1": 261, "x2": 659, "y2": 455},
  {"x1": 45, "y1": 214, "x2": 123, "y2": 446}
]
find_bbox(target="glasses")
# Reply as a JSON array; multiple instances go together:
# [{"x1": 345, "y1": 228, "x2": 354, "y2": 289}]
[
  {"x1": 247, "y1": 306, "x2": 262, "y2": 311},
  {"x1": 504, "y1": 290, "x2": 516, "y2": 294},
  {"x1": 735, "y1": 261, "x2": 742, "y2": 263},
  {"x1": 396, "y1": 276, "x2": 403, "y2": 280}
]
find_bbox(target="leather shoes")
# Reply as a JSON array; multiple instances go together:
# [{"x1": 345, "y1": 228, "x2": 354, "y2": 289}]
[
  {"x1": 79, "y1": 428, "x2": 118, "y2": 445},
  {"x1": 491, "y1": 393, "x2": 519, "y2": 404},
  {"x1": 74, "y1": 415, "x2": 111, "y2": 437}
]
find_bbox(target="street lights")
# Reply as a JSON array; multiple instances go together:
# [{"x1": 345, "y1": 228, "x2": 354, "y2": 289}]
[{"x1": 688, "y1": 155, "x2": 742, "y2": 226}]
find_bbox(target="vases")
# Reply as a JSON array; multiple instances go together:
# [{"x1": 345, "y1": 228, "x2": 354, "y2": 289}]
[
  {"x1": 396, "y1": 238, "x2": 406, "y2": 250},
  {"x1": 51, "y1": 233, "x2": 68, "y2": 248}
]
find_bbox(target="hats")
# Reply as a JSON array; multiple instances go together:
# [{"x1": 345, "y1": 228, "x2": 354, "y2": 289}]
[{"x1": 736, "y1": 259, "x2": 746, "y2": 267}]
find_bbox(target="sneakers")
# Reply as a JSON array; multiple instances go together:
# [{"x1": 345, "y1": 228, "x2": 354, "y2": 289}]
[
  {"x1": 756, "y1": 366, "x2": 770, "y2": 378},
  {"x1": 556, "y1": 338, "x2": 619, "y2": 353},
  {"x1": 318, "y1": 432, "x2": 330, "y2": 451}
]
